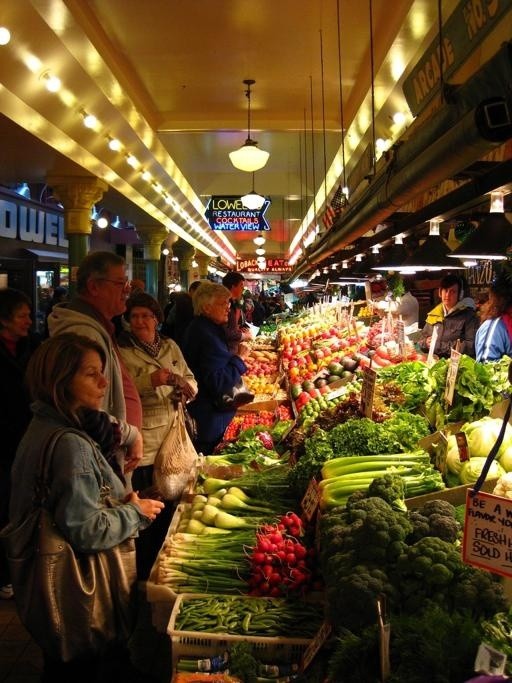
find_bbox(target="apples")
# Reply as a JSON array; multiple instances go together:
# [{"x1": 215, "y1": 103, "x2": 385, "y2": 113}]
[
  {"x1": 360, "y1": 334, "x2": 409, "y2": 369},
  {"x1": 295, "y1": 386, "x2": 332, "y2": 414},
  {"x1": 278, "y1": 313, "x2": 369, "y2": 377},
  {"x1": 246, "y1": 363, "x2": 272, "y2": 375}
]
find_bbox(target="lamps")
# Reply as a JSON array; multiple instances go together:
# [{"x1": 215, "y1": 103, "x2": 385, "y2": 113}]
[
  {"x1": 240, "y1": 171, "x2": 266, "y2": 209},
  {"x1": 0, "y1": 26, "x2": 173, "y2": 206},
  {"x1": 253, "y1": 234, "x2": 266, "y2": 269},
  {"x1": 229, "y1": 78, "x2": 271, "y2": 172}
]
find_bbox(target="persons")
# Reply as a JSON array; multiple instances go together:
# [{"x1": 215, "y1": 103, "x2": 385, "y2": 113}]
[
  {"x1": 47, "y1": 250, "x2": 143, "y2": 491},
  {"x1": 9, "y1": 332, "x2": 166, "y2": 681},
  {"x1": 41, "y1": 284, "x2": 67, "y2": 331},
  {"x1": 416, "y1": 274, "x2": 479, "y2": 359},
  {"x1": 473, "y1": 277, "x2": 512, "y2": 362},
  {"x1": 0, "y1": 286, "x2": 32, "y2": 598},
  {"x1": 368, "y1": 278, "x2": 419, "y2": 327},
  {"x1": 120, "y1": 273, "x2": 281, "y2": 491}
]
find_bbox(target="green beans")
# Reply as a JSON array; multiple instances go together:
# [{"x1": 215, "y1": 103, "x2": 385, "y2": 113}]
[{"x1": 174, "y1": 597, "x2": 322, "y2": 639}]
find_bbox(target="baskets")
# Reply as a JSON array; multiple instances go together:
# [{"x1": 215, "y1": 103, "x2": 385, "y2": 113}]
[{"x1": 167, "y1": 591, "x2": 315, "y2": 668}]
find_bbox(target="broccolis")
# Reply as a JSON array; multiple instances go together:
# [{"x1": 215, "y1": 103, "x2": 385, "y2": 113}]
[{"x1": 319, "y1": 474, "x2": 510, "y2": 636}]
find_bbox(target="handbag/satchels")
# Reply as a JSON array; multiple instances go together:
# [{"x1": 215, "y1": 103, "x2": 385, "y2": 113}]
[
  {"x1": 210, "y1": 375, "x2": 256, "y2": 412},
  {"x1": 0, "y1": 499, "x2": 148, "y2": 664},
  {"x1": 155, "y1": 418, "x2": 201, "y2": 499}
]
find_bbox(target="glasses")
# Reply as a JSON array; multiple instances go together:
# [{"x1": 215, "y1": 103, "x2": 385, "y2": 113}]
[
  {"x1": 215, "y1": 303, "x2": 232, "y2": 310},
  {"x1": 128, "y1": 314, "x2": 153, "y2": 321},
  {"x1": 98, "y1": 275, "x2": 132, "y2": 289}
]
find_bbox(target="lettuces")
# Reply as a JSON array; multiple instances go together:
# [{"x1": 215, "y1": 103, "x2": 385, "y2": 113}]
[{"x1": 288, "y1": 413, "x2": 431, "y2": 498}]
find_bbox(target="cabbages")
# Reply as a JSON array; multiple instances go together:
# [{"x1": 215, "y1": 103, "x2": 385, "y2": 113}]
[{"x1": 432, "y1": 417, "x2": 512, "y2": 484}]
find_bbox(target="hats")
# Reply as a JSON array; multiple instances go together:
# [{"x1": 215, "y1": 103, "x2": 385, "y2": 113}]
[{"x1": 123, "y1": 290, "x2": 165, "y2": 322}]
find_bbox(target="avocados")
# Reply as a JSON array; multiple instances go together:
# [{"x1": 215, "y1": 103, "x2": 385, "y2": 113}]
[{"x1": 291, "y1": 350, "x2": 375, "y2": 400}]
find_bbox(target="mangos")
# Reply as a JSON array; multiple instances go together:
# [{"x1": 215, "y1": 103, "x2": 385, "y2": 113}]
[{"x1": 244, "y1": 351, "x2": 278, "y2": 368}]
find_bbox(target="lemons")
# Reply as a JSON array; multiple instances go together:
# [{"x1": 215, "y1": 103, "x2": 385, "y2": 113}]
[
  {"x1": 358, "y1": 307, "x2": 372, "y2": 317},
  {"x1": 242, "y1": 374, "x2": 280, "y2": 393}
]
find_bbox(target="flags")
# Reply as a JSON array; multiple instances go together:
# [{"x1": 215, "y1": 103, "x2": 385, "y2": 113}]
[{"x1": 323, "y1": 183, "x2": 343, "y2": 230}]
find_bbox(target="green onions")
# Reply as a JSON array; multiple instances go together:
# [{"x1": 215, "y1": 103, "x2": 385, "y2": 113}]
[{"x1": 158, "y1": 532, "x2": 257, "y2": 593}]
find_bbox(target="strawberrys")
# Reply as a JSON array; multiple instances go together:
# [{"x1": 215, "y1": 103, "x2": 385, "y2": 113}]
[{"x1": 223, "y1": 405, "x2": 290, "y2": 451}]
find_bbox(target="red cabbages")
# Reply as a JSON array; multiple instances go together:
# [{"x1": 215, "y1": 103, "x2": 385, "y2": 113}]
[{"x1": 370, "y1": 280, "x2": 387, "y2": 292}]
